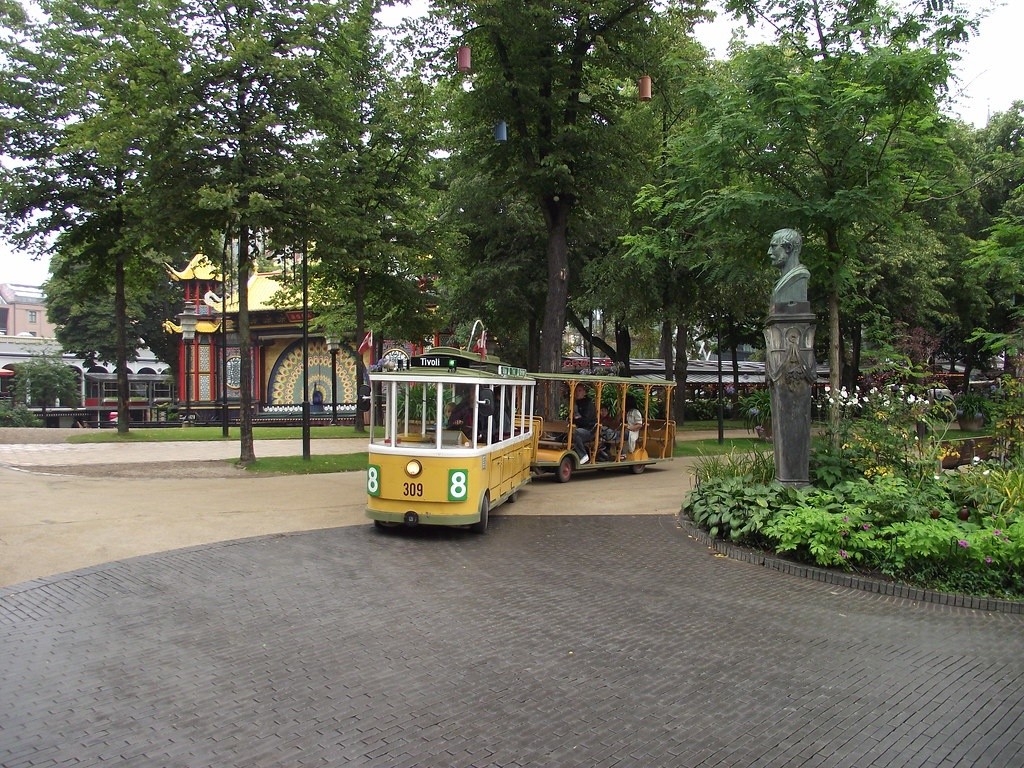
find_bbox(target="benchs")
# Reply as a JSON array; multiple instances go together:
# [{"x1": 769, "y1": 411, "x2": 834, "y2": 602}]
[
  {"x1": 513, "y1": 413, "x2": 676, "y2": 465},
  {"x1": 424, "y1": 420, "x2": 529, "y2": 446}
]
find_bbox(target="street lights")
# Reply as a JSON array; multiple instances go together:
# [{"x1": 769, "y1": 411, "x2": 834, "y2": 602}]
[{"x1": 709, "y1": 303, "x2": 724, "y2": 445}]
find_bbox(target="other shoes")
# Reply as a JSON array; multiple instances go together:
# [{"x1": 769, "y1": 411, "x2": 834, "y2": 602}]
[{"x1": 620, "y1": 453, "x2": 626, "y2": 460}]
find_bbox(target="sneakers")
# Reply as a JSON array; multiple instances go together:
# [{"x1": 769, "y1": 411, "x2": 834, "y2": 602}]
[{"x1": 580, "y1": 454, "x2": 589, "y2": 464}]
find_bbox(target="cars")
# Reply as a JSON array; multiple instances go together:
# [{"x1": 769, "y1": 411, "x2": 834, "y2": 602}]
[{"x1": 926, "y1": 382, "x2": 953, "y2": 401}]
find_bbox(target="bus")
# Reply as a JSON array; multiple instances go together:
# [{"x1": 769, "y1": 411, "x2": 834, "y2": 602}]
[{"x1": 365, "y1": 347, "x2": 677, "y2": 534}]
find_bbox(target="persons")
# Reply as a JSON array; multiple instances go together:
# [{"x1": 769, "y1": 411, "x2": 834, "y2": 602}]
[
  {"x1": 493, "y1": 386, "x2": 514, "y2": 434},
  {"x1": 597, "y1": 404, "x2": 617, "y2": 463},
  {"x1": 448, "y1": 383, "x2": 486, "y2": 444},
  {"x1": 433, "y1": 402, "x2": 457, "y2": 427},
  {"x1": 616, "y1": 394, "x2": 644, "y2": 461},
  {"x1": 556, "y1": 383, "x2": 597, "y2": 465},
  {"x1": 769, "y1": 228, "x2": 811, "y2": 302}
]
